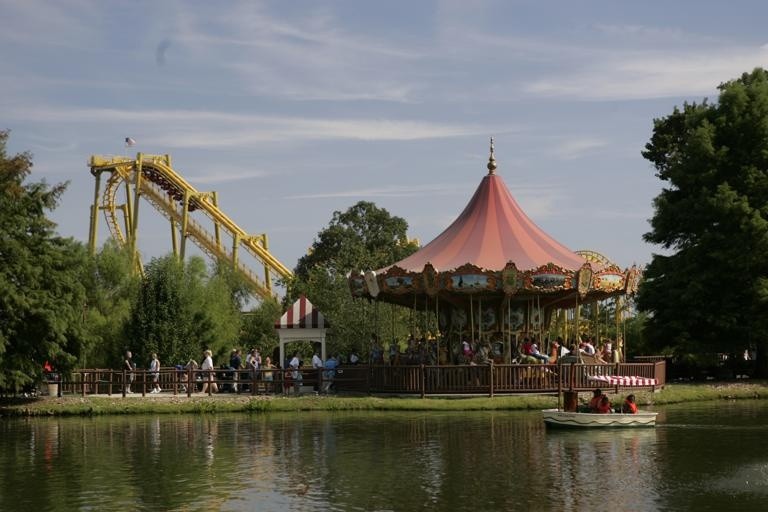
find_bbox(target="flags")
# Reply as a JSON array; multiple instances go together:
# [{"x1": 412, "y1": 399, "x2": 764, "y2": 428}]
[{"x1": 126, "y1": 137, "x2": 136, "y2": 147}]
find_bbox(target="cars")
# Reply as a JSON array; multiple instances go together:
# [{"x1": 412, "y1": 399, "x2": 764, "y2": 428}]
[{"x1": 723, "y1": 359, "x2": 755, "y2": 378}]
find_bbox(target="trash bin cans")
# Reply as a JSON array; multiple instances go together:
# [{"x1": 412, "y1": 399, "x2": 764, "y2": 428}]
[{"x1": 46, "y1": 380, "x2": 59, "y2": 397}]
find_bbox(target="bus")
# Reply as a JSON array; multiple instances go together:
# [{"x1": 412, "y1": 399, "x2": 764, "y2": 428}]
[{"x1": 634, "y1": 355, "x2": 676, "y2": 363}]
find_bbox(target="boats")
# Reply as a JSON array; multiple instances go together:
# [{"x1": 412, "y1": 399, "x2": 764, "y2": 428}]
[{"x1": 542, "y1": 408, "x2": 659, "y2": 428}]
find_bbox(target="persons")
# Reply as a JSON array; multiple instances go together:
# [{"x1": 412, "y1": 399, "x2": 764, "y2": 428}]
[
  {"x1": 351, "y1": 332, "x2": 624, "y2": 376},
  {"x1": 174, "y1": 347, "x2": 341, "y2": 395},
  {"x1": 123, "y1": 350, "x2": 135, "y2": 394},
  {"x1": 585, "y1": 389, "x2": 638, "y2": 413},
  {"x1": 149, "y1": 352, "x2": 162, "y2": 394}
]
